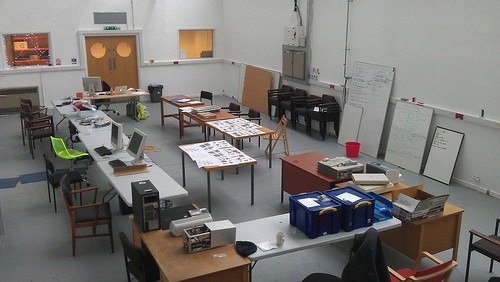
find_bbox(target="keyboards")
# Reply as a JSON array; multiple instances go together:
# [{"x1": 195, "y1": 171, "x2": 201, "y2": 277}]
[{"x1": 94, "y1": 146, "x2": 112, "y2": 156}]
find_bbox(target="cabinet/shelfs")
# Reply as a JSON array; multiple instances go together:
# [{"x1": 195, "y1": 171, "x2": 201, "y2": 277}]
[{"x1": 130, "y1": 204, "x2": 251, "y2": 282}]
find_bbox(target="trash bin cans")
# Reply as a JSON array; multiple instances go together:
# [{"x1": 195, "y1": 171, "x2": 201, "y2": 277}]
[{"x1": 148, "y1": 85, "x2": 163, "y2": 103}]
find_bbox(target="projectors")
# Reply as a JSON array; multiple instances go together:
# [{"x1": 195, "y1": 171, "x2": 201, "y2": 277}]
[{"x1": 317, "y1": 156, "x2": 365, "y2": 180}]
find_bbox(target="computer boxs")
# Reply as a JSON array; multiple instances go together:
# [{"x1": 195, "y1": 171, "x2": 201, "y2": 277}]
[
  {"x1": 131, "y1": 180, "x2": 160, "y2": 232},
  {"x1": 109, "y1": 159, "x2": 127, "y2": 168}
]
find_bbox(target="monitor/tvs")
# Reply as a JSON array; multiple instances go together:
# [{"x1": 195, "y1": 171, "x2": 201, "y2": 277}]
[
  {"x1": 110, "y1": 121, "x2": 124, "y2": 152},
  {"x1": 126, "y1": 128, "x2": 157, "y2": 167},
  {"x1": 82, "y1": 77, "x2": 102, "y2": 95}
]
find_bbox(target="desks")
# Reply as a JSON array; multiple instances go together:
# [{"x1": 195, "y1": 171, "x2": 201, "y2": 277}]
[
  {"x1": 51, "y1": 89, "x2": 275, "y2": 213},
  {"x1": 335, "y1": 181, "x2": 464, "y2": 262},
  {"x1": 235, "y1": 212, "x2": 402, "y2": 282},
  {"x1": 279, "y1": 151, "x2": 352, "y2": 203}
]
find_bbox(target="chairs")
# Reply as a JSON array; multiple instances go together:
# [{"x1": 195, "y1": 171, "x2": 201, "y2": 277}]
[
  {"x1": 268, "y1": 84, "x2": 340, "y2": 140},
  {"x1": 465, "y1": 218, "x2": 500, "y2": 282},
  {"x1": 190, "y1": 91, "x2": 212, "y2": 127},
  {"x1": 231, "y1": 108, "x2": 262, "y2": 150},
  {"x1": 19, "y1": 81, "x2": 120, "y2": 257},
  {"x1": 214, "y1": 103, "x2": 241, "y2": 136},
  {"x1": 387, "y1": 251, "x2": 458, "y2": 282},
  {"x1": 119, "y1": 232, "x2": 153, "y2": 282},
  {"x1": 263, "y1": 115, "x2": 289, "y2": 158},
  {"x1": 301, "y1": 227, "x2": 378, "y2": 282}
]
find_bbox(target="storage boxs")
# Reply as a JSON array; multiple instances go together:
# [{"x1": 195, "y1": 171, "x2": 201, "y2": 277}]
[
  {"x1": 290, "y1": 186, "x2": 376, "y2": 239},
  {"x1": 392, "y1": 184, "x2": 449, "y2": 222}
]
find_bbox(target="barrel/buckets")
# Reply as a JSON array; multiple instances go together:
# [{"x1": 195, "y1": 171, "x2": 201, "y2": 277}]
[{"x1": 345, "y1": 142, "x2": 362, "y2": 157}]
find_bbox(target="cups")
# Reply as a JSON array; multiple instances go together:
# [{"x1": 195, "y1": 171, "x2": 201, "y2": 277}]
[{"x1": 276, "y1": 232, "x2": 286, "y2": 246}]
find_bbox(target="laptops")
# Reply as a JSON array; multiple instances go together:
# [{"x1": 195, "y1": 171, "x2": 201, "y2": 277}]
[{"x1": 114, "y1": 86, "x2": 127, "y2": 95}]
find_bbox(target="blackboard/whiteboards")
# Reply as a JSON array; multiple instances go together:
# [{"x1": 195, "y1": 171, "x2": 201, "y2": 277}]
[
  {"x1": 385, "y1": 102, "x2": 433, "y2": 174},
  {"x1": 338, "y1": 104, "x2": 363, "y2": 147},
  {"x1": 423, "y1": 126, "x2": 465, "y2": 185},
  {"x1": 336, "y1": 61, "x2": 396, "y2": 159},
  {"x1": 237, "y1": 64, "x2": 280, "y2": 117}
]
